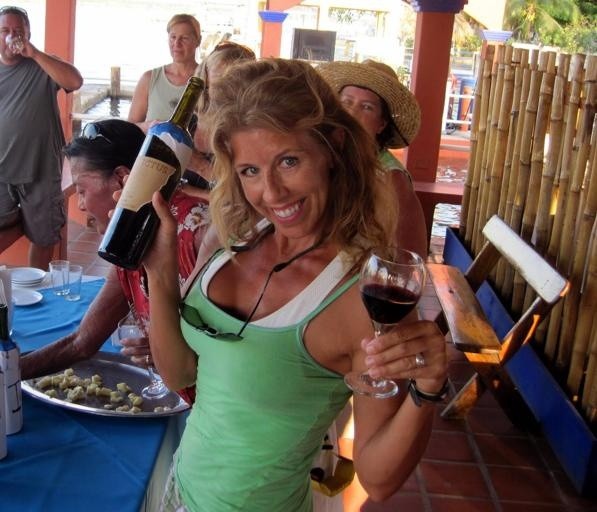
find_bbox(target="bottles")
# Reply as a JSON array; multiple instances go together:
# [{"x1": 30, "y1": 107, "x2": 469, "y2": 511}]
[
  {"x1": 97, "y1": 78, "x2": 205, "y2": 271},
  {"x1": 0, "y1": 304, "x2": 22, "y2": 436}
]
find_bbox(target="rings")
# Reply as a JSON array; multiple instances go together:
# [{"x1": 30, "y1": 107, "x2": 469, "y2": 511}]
[{"x1": 414, "y1": 355, "x2": 434, "y2": 372}]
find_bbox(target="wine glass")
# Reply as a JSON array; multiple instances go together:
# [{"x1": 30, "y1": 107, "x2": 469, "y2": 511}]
[
  {"x1": 5, "y1": 34, "x2": 24, "y2": 53},
  {"x1": 118, "y1": 312, "x2": 171, "y2": 400},
  {"x1": 345, "y1": 247, "x2": 426, "y2": 399}
]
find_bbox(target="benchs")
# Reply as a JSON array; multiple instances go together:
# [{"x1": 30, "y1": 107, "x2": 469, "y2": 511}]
[{"x1": 420, "y1": 214, "x2": 572, "y2": 431}]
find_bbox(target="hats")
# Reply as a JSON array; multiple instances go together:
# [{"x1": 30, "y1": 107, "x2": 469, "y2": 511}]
[{"x1": 314, "y1": 59, "x2": 421, "y2": 149}]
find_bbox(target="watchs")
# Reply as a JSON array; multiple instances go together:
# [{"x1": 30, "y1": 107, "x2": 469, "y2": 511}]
[{"x1": 408, "y1": 379, "x2": 453, "y2": 405}]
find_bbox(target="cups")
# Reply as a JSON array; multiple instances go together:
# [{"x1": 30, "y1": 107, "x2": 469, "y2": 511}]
[
  {"x1": 62, "y1": 266, "x2": 82, "y2": 301},
  {"x1": 49, "y1": 260, "x2": 70, "y2": 295}
]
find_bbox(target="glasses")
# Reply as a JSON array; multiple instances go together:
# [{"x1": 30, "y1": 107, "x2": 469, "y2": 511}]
[
  {"x1": 177, "y1": 247, "x2": 285, "y2": 342},
  {"x1": 81, "y1": 122, "x2": 113, "y2": 143},
  {"x1": 0, "y1": 6, "x2": 28, "y2": 16},
  {"x1": 215, "y1": 41, "x2": 255, "y2": 61}
]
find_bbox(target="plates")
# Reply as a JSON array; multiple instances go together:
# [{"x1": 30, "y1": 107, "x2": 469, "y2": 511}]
[
  {"x1": 11, "y1": 289, "x2": 42, "y2": 306},
  {"x1": 7, "y1": 266, "x2": 47, "y2": 288}
]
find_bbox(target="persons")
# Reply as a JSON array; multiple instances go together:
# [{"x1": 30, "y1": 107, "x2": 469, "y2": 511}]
[
  {"x1": 14, "y1": 118, "x2": 214, "y2": 407},
  {"x1": 114, "y1": 61, "x2": 447, "y2": 512},
  {"x1": 126, "y1": 16, "x2": 202, "y2": 138},
  {"x1": 0, "y1": 6, "x2": 82, "y2": 269},
  {"x1": 315, "y1": 59, "x2": 431, "y2": 279},
  {"x1": 192, "y1": 41, "x2": 255, "y2": 153}
]
